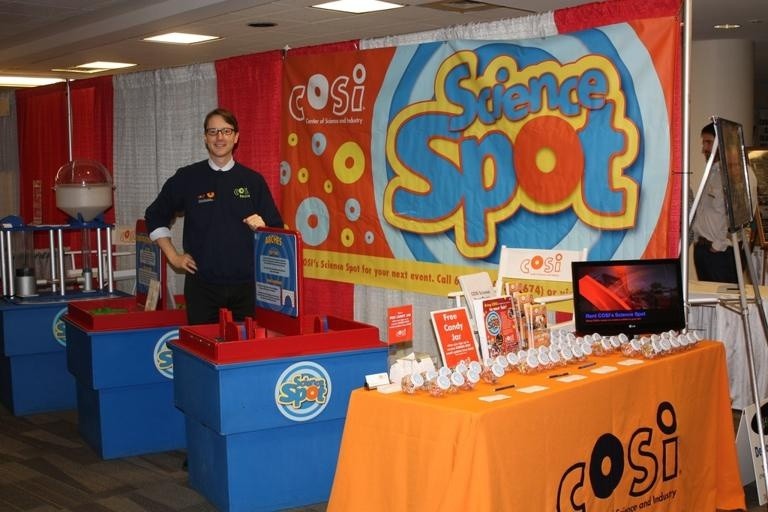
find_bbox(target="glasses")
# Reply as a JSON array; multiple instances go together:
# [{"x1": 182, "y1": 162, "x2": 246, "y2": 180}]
[{"x1": 205, "y1": 127, "x2": 237, "y2": 136}]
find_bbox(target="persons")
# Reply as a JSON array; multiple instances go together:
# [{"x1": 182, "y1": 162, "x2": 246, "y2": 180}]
[
  {"x1": 691, "y1": 124, "x2": 758, "y2": 283},
  {"x1": 143, "y1": 107, "x2": 284, "y2": 326}
]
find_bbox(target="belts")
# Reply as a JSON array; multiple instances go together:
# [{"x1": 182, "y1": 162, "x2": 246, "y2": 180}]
[{"x1": 698, "y1": 238, "x2": 713, "y2": 247}]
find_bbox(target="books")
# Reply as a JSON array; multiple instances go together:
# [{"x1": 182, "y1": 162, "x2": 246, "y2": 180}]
[
  {"x1": 474, "y1": 295, "x2": 521, "y2": 363},
  {"x1": 143, "y1": 278, "x2": 160, "y2": 312},
  {"x1": 511, "y1": 292, "x2": 535, "y2": 350},
  {"x1": 429, "y1": 306, "x2": 483, "y2": 376},
  {"x1": 457, "y1": 271, "x2": 496, "y2": 345},
  {"x1": 522, "y1": 300, "x2": 550, "y2": 351}
]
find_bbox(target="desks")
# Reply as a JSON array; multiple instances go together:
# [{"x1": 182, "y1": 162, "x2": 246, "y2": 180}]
[
  {"x1": 326, "y1": 334, "x2": 750, "y2": 512},
  {"x1": 688, "y1": 279, "x2": 768, "y2": 410}
]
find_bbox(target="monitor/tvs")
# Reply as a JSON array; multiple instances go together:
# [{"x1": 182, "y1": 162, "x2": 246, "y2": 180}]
[{"x1": 572, "y1": 258, "x2": 685, "y2": 337}]
